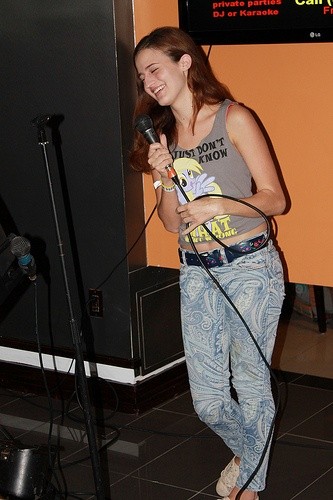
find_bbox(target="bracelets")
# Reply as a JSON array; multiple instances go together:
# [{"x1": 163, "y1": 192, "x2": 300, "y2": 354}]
[{"x1": 160, "y1": 180, "x2": 175, "y2": 192}]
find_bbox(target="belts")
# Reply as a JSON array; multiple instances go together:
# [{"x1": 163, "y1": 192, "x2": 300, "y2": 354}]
[{"x1": 176, "y1": 235, "x2": 268, "y2": 269}]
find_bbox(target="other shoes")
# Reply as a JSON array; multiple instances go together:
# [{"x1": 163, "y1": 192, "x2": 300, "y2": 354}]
[
  {"x1": 218, "y1": 495, "x2": 259, "y2": 500},
  {"x1": 217, "y1": 453, "x2": 240, "y2": 496}
]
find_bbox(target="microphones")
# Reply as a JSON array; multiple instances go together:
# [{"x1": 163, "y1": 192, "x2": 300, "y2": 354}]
[
  {"x1": 11, "y1": 236, "x2": 38, "y2": 286},
  {"x1": 133, "y1": 114, "x2": 179, "y2": 185}
]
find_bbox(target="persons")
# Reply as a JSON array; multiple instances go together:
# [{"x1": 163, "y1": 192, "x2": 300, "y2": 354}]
[{"x1": 131, "y1": 26, "x2": 286, "y2": 500}]
